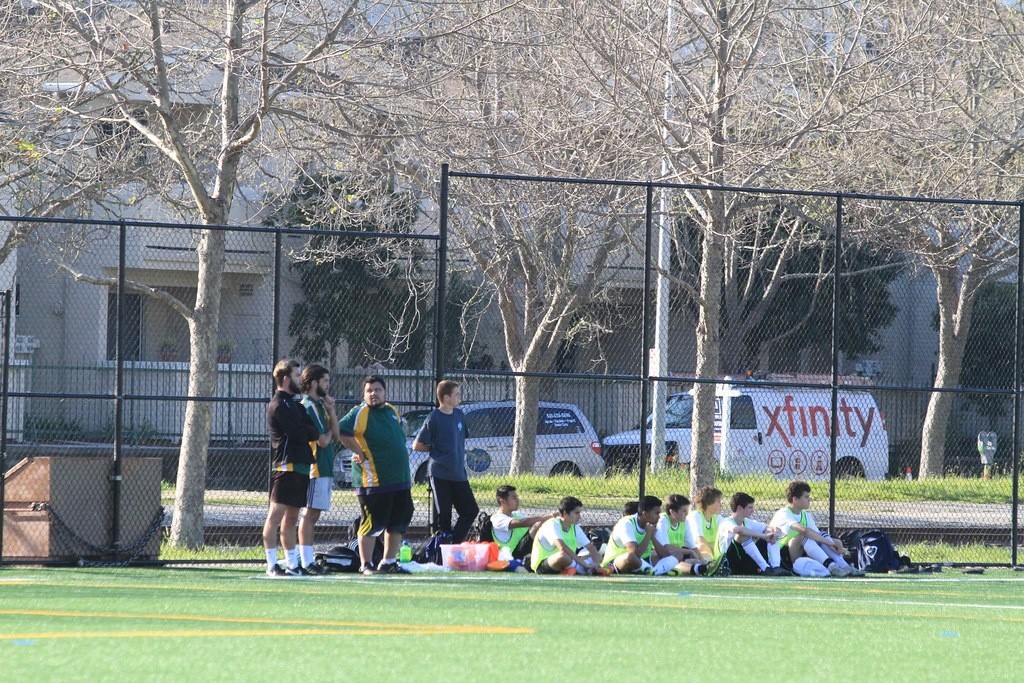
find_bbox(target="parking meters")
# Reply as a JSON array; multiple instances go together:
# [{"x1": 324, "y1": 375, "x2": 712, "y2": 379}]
[{"x1": 977, "y1": 431, "x2": 998, "y2": 477}]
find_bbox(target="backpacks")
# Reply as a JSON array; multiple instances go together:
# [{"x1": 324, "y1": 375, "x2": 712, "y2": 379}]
[
  {"x1": 588, "y1": 528, "x2": 612, "y2": 557},
  {"x1": 839, "y1": 530, "x2": 894, "y2": 574},
  {"x1": 465, "y1": 511, "x2": 493, "y2": 543}
]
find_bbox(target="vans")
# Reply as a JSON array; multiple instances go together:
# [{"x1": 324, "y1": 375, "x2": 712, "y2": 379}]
[{"x1": 603, "y1": 375, "x2": 890, "y2": 482}]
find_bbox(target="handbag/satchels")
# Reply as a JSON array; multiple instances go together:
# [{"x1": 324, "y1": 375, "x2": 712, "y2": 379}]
[
  {"x1": 411, "y1": 530, "x2": 453, "y2": 567},
  {"x1": 315, "y1": 546, "x2": 361, "y2": 573}
]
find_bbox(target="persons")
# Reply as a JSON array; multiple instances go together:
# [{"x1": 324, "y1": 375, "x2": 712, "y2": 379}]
[
  {"x1": 413, "y1": 380, "x2": 480, "y2": 546},
  {"x1": 261, "y1": 358, "x2": 321, "y2": 577},
  {"x1": 977, "y1": 423, "x2": 998, "y2": 480},
  {"x1": 487, "y1": 483, "x2": 862, "y2": 578},
  {"x1": 296, "y1": 363, "x2": 339, "y2": 576},
  {"x1": 336, "y1": 373, "x2": 415, "y2": 576}
]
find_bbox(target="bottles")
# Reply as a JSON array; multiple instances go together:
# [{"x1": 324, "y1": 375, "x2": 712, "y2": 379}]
[
  {"x1": 905, "y1": 467, "x2": 912, "y2": 481},
  {"x1": 400, "y1": 541, "x2": 412, "y2": 563}
]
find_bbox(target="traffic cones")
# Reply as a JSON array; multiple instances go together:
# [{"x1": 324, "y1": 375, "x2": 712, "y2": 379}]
[{"x1": 905, "y1": 467, "x2": 912, "y2": 481}]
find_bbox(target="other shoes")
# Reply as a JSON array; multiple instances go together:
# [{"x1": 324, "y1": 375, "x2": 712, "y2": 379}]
[{"x1": 896, "y1": 563, "x2": 942, "y2": 574}]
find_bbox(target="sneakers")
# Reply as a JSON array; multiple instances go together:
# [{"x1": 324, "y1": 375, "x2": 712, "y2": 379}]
[
  {"x1": 560, "y1": 567, "x2": 576, "y2": 576},
  {"x1": 359, "y1": 561, "x2": 386, "y2": 575},
  {"x1": 829, "y1": 563, "x2": 865, "y2": 578},
  {"x1": 660, "y1": 568, "x2": 682, "y2": 578},
  {"x1": 755, "y1": 567, "x2": 793, "y2": 577},
  {"x1": 703, "y1": 554, "x2": 731, "y2": 578},
  {"x1": 266, "y1": 564, "x2": 291, "y2": 577},
  {"x1": 598, "y1": 566, "x2": 615, "y2": 576},
  {"x1": 632, "y1": 567, "x2": 653, "y2": 575},
  {"x1": 377, "y1": 561, "x2": 412, "y2": 575},
  {"x1": 301, "y1": 562, "x2": 331, "y2": 575},
  {"x1": 285, "y1": 563, "x2": 315, "y2": 576}
]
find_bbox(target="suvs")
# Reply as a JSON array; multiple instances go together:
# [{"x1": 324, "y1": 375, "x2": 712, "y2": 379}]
[{"x1": 332, "y1": 399, "x2": 605, "y2": 487}]
